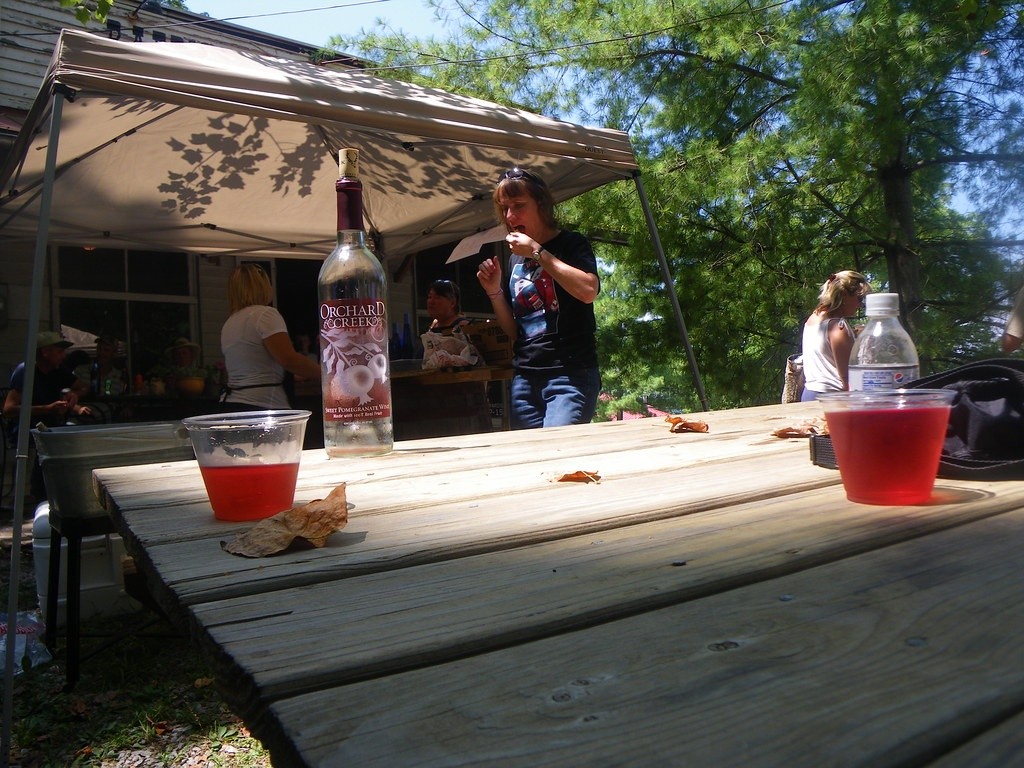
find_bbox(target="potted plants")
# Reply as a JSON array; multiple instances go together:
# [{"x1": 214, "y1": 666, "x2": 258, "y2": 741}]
[
  {"x1": 176, "y1": 367, "x2": 207, "y2": 396},
  {"x1": 200, "y1": 378, "x2": 224, "y2": 401}
]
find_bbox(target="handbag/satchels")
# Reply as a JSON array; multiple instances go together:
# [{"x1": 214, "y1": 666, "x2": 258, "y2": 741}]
[
  {"x1": 810, "y1": 358, "x2": 1024, "y2": 476},
  {"x1": 780, "y1": 353, "x2": 805, "y2": 404}
]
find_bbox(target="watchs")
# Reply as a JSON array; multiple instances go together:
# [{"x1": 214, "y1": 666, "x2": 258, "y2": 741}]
[{"x1": 533, "y1": 246, "x2": 544, "y2": 261}]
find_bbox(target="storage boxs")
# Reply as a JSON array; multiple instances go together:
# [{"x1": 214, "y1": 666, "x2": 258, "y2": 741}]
[{"x1": 32, "y1": 501, "x2": 125, "y2": 626}]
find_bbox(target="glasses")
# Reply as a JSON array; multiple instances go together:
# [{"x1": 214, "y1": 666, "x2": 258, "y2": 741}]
[
  {"x1": 435, "y1": 278, "x2": 456, "y2": 294},
  {"x1": 495, "y1": 167, "x2": 544, "y2": 191}
]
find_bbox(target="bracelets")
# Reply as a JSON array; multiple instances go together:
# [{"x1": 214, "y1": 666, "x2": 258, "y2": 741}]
[{"x1": 487, "y1": 289, "x2": 503, "y2": 300}]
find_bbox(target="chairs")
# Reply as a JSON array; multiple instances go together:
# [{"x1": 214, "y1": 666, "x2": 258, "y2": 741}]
[{"x1": 0, "y1": 387, "x2": 37, "y2": 498}]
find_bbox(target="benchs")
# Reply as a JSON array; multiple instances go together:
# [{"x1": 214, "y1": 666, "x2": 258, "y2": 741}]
[{"x1": 42, "y1": 510, "x2": 120, "y2": 694}]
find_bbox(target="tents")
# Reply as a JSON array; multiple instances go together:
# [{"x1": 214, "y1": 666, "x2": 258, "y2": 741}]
[{"x1": 0, "y1": 24, "x2": 713, "y2": 768}]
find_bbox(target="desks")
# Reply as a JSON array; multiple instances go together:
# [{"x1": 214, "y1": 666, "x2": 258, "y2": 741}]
[
  {"x1": 93, "y1": 402, "x2": 1024, "y2": 768},
  {"x1": 292, "y1": 358, "x2": 515, "y2": 449}
]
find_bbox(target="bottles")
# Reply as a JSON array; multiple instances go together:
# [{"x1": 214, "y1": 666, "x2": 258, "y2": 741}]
[
  {"x1": 400, "y1": 312, "x2": 414, "y2": 359},
  {"x1": 847, "y1": 293, "x2": 920, "y2": 391},
  {"x1": 317, "y1": 176, "x2": 394, "y2": 458},
  {"x1": 389, "y1": 321, "x2": 401, "y2": 361}
]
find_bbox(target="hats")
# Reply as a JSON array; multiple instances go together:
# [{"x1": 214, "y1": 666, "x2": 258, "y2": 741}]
[
  {"x1": 94, "y1": 333, "x2": 119, "y2": 346},
  {"x1": 37, "y1": 331, "x2": 74, "y2": 349},
  {"x1": 163, "y1": 336, "x2": 201, "y2": 362}
]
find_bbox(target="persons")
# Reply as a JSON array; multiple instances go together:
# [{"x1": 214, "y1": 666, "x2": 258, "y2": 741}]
[
  {"x1": 415, "y1": 280, "x2": 479, "y2": 357},
  {"x1": 799, "y1": 270, "x2": 870, "y2": 404},
  {"x1": 1002, "y1": 286, "x2": 1024, "y2": 352},
  {"x1": 476, "y1": 169, "x2": 602, "y2": 427},
  {"x1": 219, "y1": 263, "x2": 322, "y2": 412},
  {"x1": 4, "y1": 331, "x2": 205, "y2": 492}
]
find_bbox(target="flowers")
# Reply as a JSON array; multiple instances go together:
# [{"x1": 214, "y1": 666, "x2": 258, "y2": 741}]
[{"x1": 206, "y1": 360, "x2": 230, "y2": 385}]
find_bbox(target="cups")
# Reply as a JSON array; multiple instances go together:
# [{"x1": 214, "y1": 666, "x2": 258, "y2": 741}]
[
  {"x1": 819, "y1": 390, "x2": 956, "y2": 506},
  {"x1": 181, "y1": 409, "x2": 312, "y2": 522}
]
